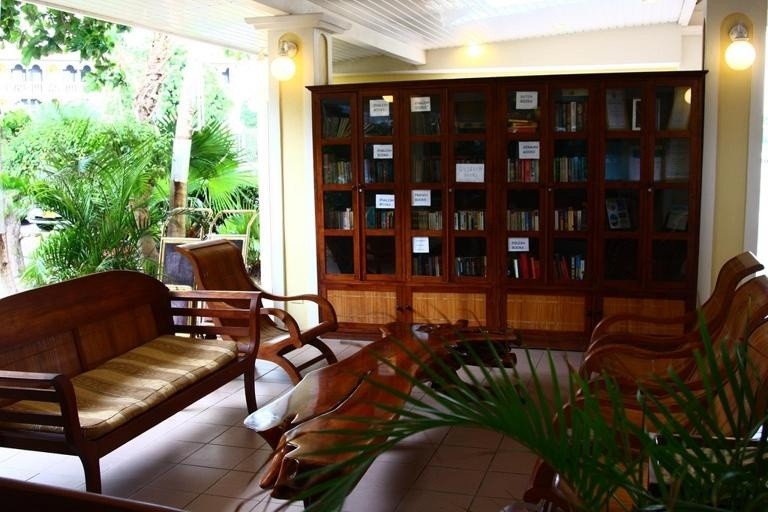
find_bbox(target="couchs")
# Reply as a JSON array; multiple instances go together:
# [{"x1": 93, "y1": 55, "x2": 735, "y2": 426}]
[{"x1": 0, "y1": 270, "x2": 263, "y2": 496}]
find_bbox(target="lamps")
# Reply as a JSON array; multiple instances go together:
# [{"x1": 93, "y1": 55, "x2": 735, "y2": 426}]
[
  {"x1": 269, "y1": 39, "x2": 298, "y2": 81},
  {"x1": 723, "y1": 22, "x2": 756, "y2": 71}
]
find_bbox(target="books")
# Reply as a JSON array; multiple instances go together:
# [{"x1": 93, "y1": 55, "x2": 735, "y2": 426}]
[{"x1": 322, "y1": 99, "x2": 585, "y2": 280}]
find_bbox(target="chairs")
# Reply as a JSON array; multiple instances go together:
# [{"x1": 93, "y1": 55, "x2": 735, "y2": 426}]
[
  {"x1": 158, "y1": 207, "x2": 213, "y2": 339},
  {"x1": 197, "y1": 209, "x2": 259, "y2": 339},
  {"x1": 176, "y1": 239, "x2": 338, "y2": 387}
]
